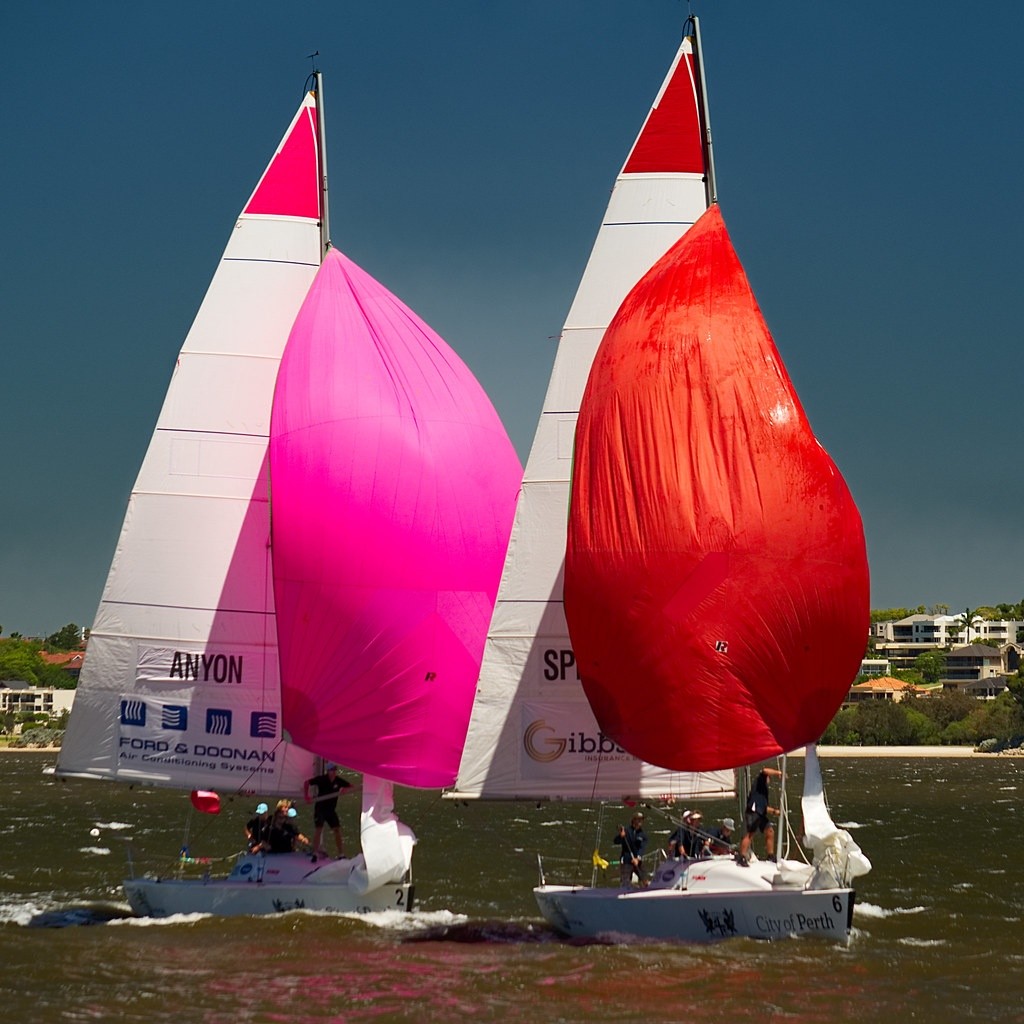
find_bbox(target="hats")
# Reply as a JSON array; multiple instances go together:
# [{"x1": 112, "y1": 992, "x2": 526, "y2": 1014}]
[
  {"x1": 683, "y1": 810, "x2": 691, "y2": 818},
  {"x1": 690, "y1": 813, "x2": 703, "y2": 819},
  {"x1": 326, "y1": 760, "x2": 337, "y2": 769},
  {"x1": 632, "y1": 812, "x2": 647, "y2": 820},
  {"x1": 287, "y1": 808, "x2": 297, "y2": 817},
  {"x1": 255, "y1": 802, "x2": 268, "y2": 814},
  {"x1": 723, "y1": 818, "x2": 736, "y2": 831}
]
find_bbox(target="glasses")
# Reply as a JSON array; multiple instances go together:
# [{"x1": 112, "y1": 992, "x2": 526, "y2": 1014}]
[{"x1": 327, "y1": 768, "x2": 337, "y2": 771}]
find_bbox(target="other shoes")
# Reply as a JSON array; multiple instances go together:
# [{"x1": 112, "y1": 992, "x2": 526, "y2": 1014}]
[
  {"x1": 766, "y1": 854, "x2": 777, "y2": 864},
  {"x1": 736, "y1": 855, "x2": 749, "y2": 867}
]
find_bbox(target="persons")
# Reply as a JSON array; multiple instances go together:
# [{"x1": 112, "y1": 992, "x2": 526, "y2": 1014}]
[
  {"x1": 613, "y1": 811, "x2": 649, "y2": 889},
  {"x1": 667, "y1": 810, "x2": 693, "y2": 857},
  {"x1": 674, "y1": 811, "x2": 710, "y2": 859},
  {"x1": 243, "y1": 799, "x2": 310, "y2": 854},
  {"x1": 739, "y1": 767, "x2": 782, "y2": 867},
  {"x1": 304, "y1": 763, "x2": 349, "y2": 863},
  {"x1": 713, "y1": 817, "x2": 736, "y2": 855}
]
